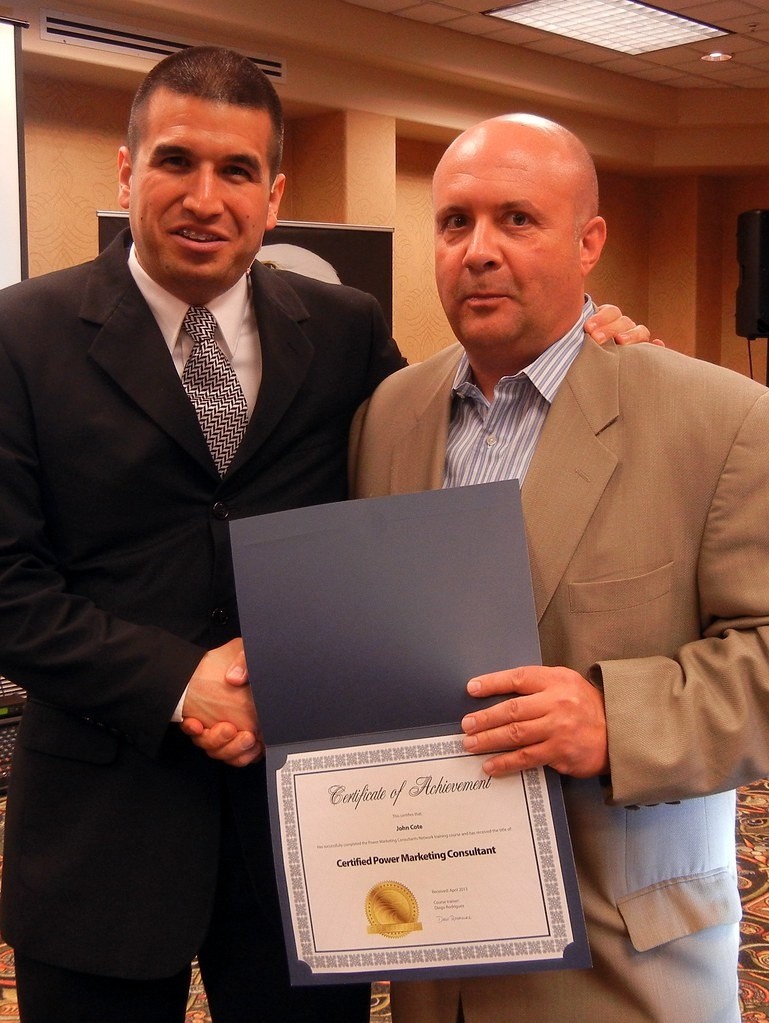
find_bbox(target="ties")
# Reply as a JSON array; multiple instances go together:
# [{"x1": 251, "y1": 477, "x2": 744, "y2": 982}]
[{"x1": 182, "y1": 306, "x2": 249, "y2": 479}]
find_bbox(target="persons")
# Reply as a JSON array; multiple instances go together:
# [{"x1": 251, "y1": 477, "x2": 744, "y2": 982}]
[
  {"x1": 1, "y1": 42, "x2": 666, "y2": 1023},
  {"x1": 170, "y1": 109, "x2": 767, "y2": 1023}
]
